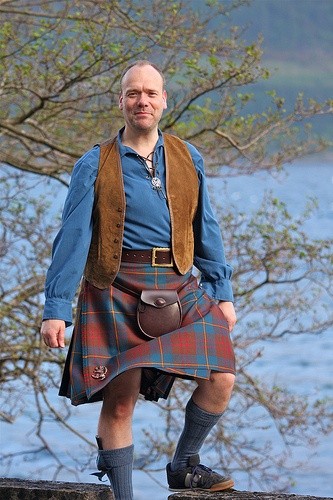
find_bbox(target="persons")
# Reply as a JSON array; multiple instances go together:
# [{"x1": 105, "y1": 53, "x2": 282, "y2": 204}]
[{"x1": 37, "y1": 62, "x2": 237, "y2": 500}]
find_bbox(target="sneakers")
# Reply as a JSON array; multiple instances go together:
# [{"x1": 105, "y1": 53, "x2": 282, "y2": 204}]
[{"x1": 166, "y1": 454, "x2": 234, "y2": 492}]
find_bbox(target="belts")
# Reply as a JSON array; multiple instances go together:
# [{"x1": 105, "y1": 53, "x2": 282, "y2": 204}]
[{"x1": 120, "y1": 247, "x2": 174, "y2": 268}]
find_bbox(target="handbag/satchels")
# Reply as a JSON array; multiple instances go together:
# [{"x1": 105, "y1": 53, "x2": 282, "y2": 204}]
[{"x1": 136, "y1": 289, "x2": 183, "y2": 338}]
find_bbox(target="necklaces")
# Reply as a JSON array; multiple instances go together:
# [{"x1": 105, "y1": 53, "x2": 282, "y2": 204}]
[{"x1": 138, "y1": 155, "x2": 164, "y2": 197}]
